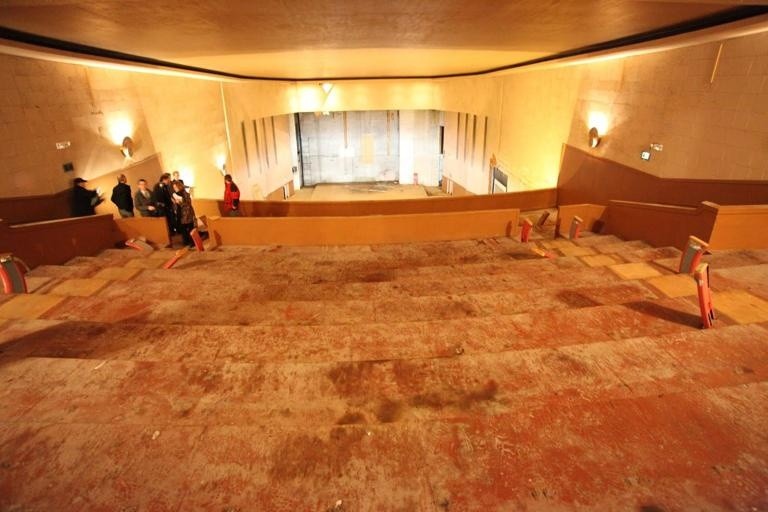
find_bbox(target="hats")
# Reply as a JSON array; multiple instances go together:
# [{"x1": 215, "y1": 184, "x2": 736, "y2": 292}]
[{"x1": 73, "y1": 178, "x2": 87, "y2": 183}]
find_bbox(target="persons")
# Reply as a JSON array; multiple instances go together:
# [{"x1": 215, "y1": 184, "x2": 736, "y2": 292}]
[
  {"x1": 69, "y1": 177, "x2": 106, "y2": 217},
  {"x1": 148, "y1": 170, "x2": 184, "y2": 237},
  {"x1": 222, "y1": 174, "x2": 240, "y2": 217},
  {"x1": 133, "y1": 178, "x2": 157, "y2": 217},
  {"x1": 170, "y1": 181, "x2": 199, "y2": 251},
  {"x1": 110, "y1": 173, "x2": 135, "y2": 218}
]
